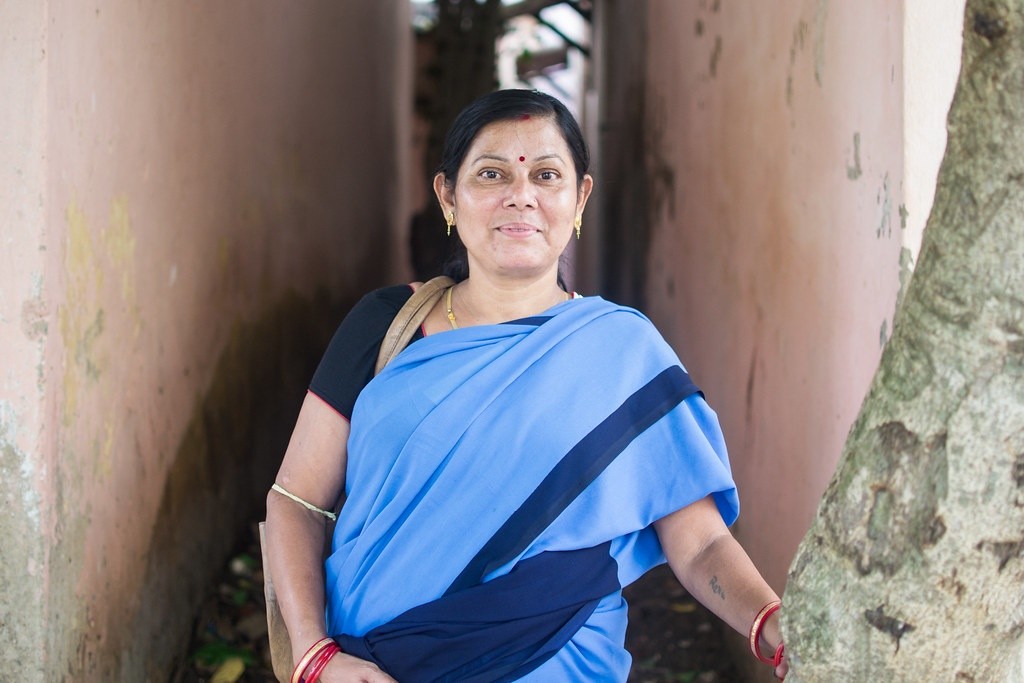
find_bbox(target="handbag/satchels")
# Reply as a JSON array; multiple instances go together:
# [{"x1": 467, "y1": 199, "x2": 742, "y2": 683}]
[{"x1": 260, "y1": 274, "x2": 452, "y2": 683}]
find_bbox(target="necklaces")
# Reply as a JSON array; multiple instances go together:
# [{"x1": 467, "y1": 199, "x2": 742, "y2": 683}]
[{"x1": 444, "y1": 279, "x2": 569, "y2": 330}]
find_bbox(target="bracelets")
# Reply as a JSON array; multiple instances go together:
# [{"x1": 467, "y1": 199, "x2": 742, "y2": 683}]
[
  {"x1": 774, "y1": 641, "x2": 787, "y2": 667},
  {"x1": 291, "y1": 636, "x2": 340, "y2": 683},
  {"x1": 748, "y1": 600, "x2": 783, "y2": 663}
]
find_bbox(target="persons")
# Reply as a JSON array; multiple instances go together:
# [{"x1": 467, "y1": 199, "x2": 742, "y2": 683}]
[{"x1": 262, "y1": 88, "x2": 784, "y2": 683}]
